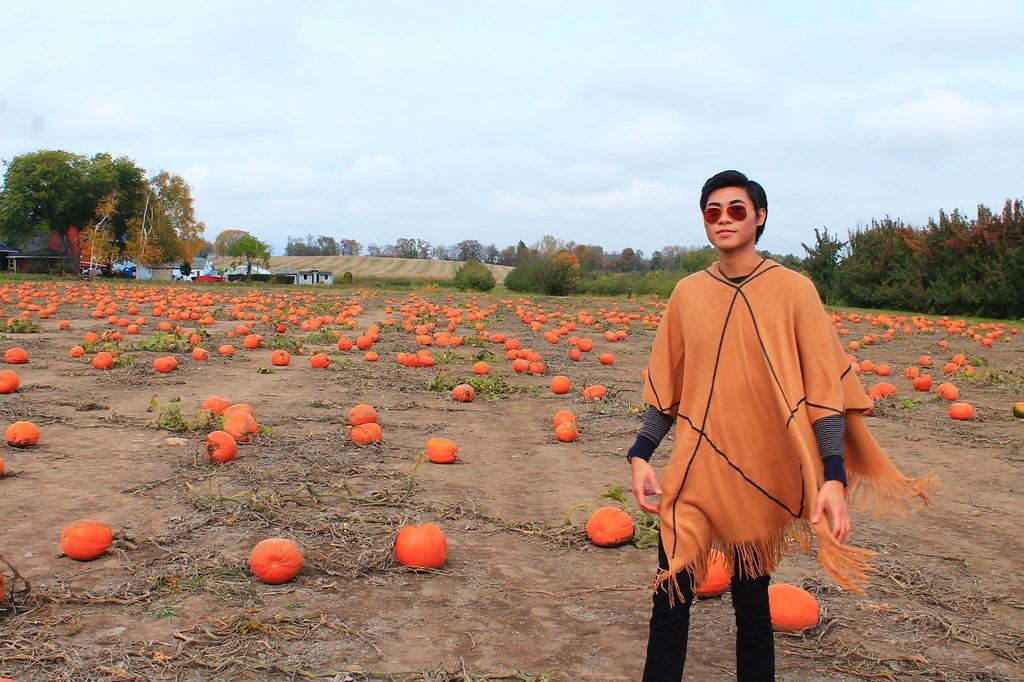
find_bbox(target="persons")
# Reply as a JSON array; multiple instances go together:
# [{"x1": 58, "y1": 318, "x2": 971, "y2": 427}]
[
  {"x1": 629, "y1": 170, "x2": 932, "y2": 681},
  {"x1": 101, "y1": 266, "x2": 106, "y2": 274}
]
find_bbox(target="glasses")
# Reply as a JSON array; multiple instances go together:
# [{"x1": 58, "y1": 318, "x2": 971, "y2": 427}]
[{"x1": 703, "y1": 205, "x2": 755, "y2": 225}]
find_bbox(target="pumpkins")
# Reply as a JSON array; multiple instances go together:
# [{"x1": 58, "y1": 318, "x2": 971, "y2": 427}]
[{"x1": 0, "y1": 281, "x2": 1024, "y2": 682}]
